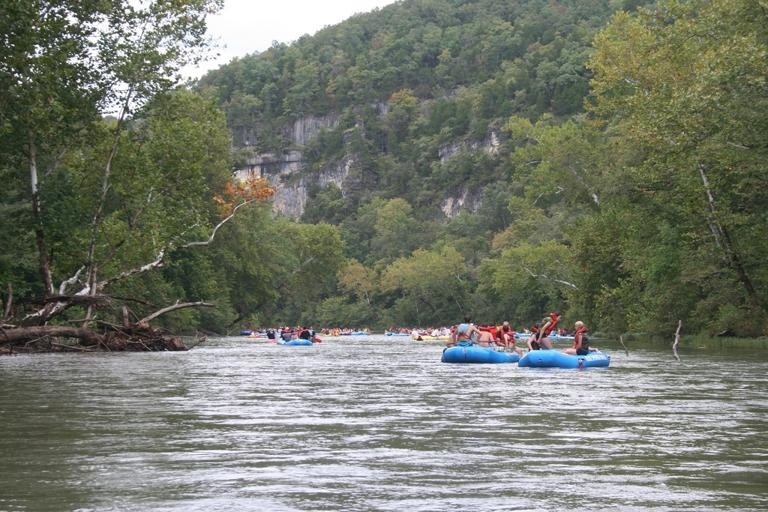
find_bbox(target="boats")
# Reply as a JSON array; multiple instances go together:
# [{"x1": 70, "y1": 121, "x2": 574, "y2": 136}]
[
  {"x1": 514, "y1": 346, "x2": 612, "y2": 371},
  {"x1": 438, "y1": 345, "x2": 521, "y2": 365}
]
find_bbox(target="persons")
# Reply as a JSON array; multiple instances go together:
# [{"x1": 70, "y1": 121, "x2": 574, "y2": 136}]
[
  {"x1": 446, "y1": 320, "x2": 522, "y2": 354},
  {"x1": 524, "y1": 310, "x2": 590, "y2": 357},
  {"x1": 320, "y1": 328, "x2": 369, "y2": 337},
  {"x1": 248, "y1": 326, "x2": 316, "y2": 344},
  {"x1": 384, "y1": 326, "x2": 450, "y2": 341}
]
